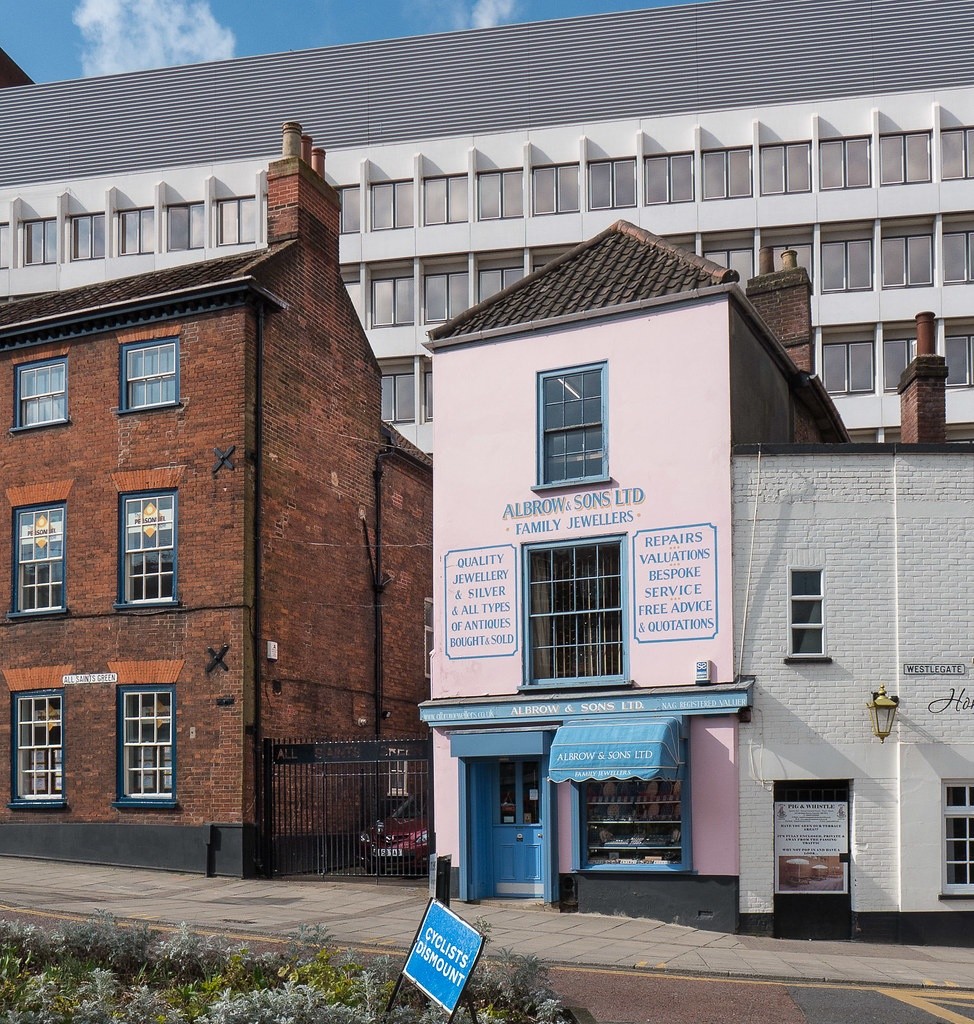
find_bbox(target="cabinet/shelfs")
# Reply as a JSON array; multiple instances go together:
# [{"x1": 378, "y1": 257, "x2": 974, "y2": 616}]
[{"x1": 586, "y1": 780, "x2": 682, "y2": 849}]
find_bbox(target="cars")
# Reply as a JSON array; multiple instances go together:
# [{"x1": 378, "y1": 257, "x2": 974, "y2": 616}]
[{"x1": 360, "y1": 792, "x2": 428, "y2": 875}]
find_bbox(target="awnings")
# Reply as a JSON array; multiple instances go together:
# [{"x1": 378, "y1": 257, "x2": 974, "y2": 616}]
[{"x1": 545, "y1": 711, "x2": 687, "y2": 783}]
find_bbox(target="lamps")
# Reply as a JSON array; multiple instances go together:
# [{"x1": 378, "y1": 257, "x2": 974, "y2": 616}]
[
  {"x1": 381, "y1": 711, "x2": 392, "y2": 720},
  {"x1": 866, "y1": 683, "x2": 899, "y2": 745}
]
